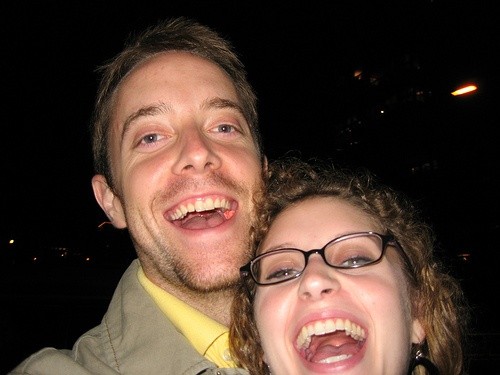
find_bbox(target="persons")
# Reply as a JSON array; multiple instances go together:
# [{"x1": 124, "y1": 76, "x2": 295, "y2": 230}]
[
  {"x1": 229, "y1": 148, "x2": 466, "y2": 375},
  {"x1": 3, "y1": 16, "x2": 267, "y2": 375}
]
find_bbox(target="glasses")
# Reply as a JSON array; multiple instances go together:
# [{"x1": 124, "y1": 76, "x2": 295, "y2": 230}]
[{"x1": 235, "y1": 230, "x2": 418, "y2": 312}]
